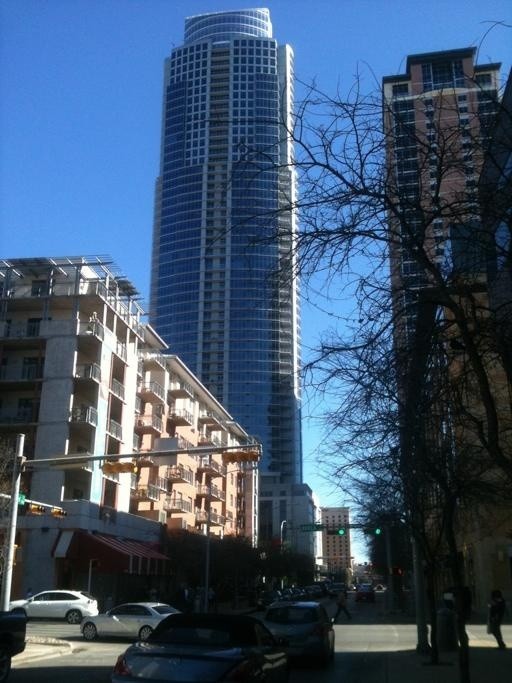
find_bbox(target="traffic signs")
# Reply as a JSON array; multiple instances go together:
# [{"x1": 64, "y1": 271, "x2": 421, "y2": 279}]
[
  {"x1": 347, "y1": 524, "x2": 362, "y2": 528},
  {"x1": 17, "y1": 493, "x2": 25, "y2": 504},
  {"x1": 301, "y1": 523, "x2": 324, "y2": 531}
]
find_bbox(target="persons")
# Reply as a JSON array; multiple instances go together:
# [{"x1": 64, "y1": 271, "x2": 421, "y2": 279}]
[
  {"x1": 334, "y1": 589, "x2": 354, "y2": 619},
  {"x1": 25, "y1": 586, "x2": 35, "y2": 599},
  {"x1": 486, "y1": 589, "x2": 506, "y2": 649},
  {"x1": 145, "y1": 584, "x2": 216, "y2": 611},
  {"x1": 441, "y1": 588, "x2": 470, "y2": 649}
]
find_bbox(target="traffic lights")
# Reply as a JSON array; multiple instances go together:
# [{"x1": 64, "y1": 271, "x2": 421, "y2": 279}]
[
  {"x1": 391, "y1": 568, "x2": 404, "y2": 575},
  {"x1": 326, "y1": 528, "x2": 346, "y2": 535},
  {"x1": 365, "y1": 527, "x2": 381, "y2": 534},
  {"x1": 30, "y1": 504, "x2": 45, "y2": 515},
  {"x1": 49, "y1": 507, "x2": 67, "y2": 519}
]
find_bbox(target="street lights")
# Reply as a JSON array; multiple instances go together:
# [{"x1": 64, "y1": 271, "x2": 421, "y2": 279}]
[{"x1": 202, "y1": 464, "x2": 257, "y2": 614}]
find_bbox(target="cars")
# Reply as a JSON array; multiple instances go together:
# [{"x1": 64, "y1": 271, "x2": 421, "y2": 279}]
[
  {"x1": 109, "y1": 608, "x2": 291, "y2": 682},
  {"x1": 78, "y1": 599, "x2": 182, "y2": 642},
  {"x1": 258, "y1": 600, "x2": 337, "y2": 671},
  {"x1": 0, "y1": 609, "x2": 28, "y2": 682},
  {"x1": 7, "y1": 587, "x2": 99, "y2": 624},
  {"x1": 253, "y1": 577, "x2": 389, "y2": 610}
]
